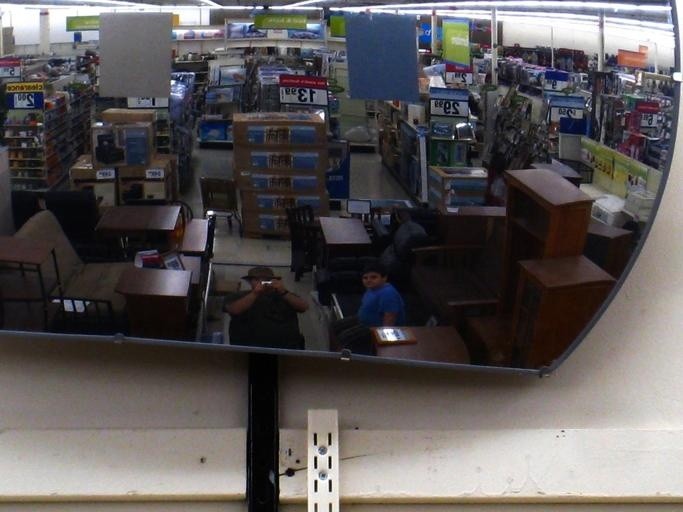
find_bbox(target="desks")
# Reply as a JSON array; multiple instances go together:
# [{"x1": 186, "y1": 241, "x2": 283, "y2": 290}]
[{"x1": 0, "y1": 201, "x2": 470, "y2": 365}]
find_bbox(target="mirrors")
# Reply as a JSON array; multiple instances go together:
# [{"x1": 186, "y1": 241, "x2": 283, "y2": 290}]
[{"x1": 0, "y1": 0, "x2": 682, "y2": 380}]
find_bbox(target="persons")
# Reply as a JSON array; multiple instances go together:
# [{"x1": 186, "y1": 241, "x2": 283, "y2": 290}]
[
  {"x1": 221, "y1": 267, "x2": 311, "y2": 351},
  {"x1": 329, "y1": 265, "x2": 406, "y2": 352},
  {"x1": 623, "y1": 220, "x2": 641, "y2": 249}
]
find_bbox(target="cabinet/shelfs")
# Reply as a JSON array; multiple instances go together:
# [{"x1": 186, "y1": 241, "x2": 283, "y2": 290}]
[
  {"x1": 1, "y1": 53, "x2": 100, "y2": 192},
  {"x1": 378, "y1": 46, "x2": 673, "y2": 204},
  {"x1": 506, "y1": 170, "x2": 596, "y2": 314},
  {"x1": 171, "y1": 46, "x2": 342, "y2": 190},
  {"x1": 512, "y1": 255, "x2": 617, "y2": 370}
]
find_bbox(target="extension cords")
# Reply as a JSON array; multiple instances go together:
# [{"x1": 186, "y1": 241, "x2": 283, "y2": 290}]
[{"x1": 307, "y1": 407, "x2": 341, "y2": 512}]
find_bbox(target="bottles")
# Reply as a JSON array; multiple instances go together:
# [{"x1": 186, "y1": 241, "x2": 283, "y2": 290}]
[{"x1": 11, "y1": 149, "x2": 23, "y2": 159}]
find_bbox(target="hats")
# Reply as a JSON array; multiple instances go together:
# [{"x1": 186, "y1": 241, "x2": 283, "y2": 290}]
[{"x1": 241, "y1": 266, "x2": 282, "y2": 280}]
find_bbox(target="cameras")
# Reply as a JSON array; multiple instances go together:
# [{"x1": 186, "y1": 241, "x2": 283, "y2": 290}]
[{"x1": 261, "y1": 280, "x2": 273, "y2": 289}]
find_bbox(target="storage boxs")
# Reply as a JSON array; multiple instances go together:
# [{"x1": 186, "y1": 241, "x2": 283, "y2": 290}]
[
  {"x1": 429, "y1": 166, "x2": 488, "y2": 206},
  {"x1": 233, "y1": 113, "x2": 330, "y2": 238},
  {"x1": 69, "y1": 109, "x2": 179, "y2": 206}
]
